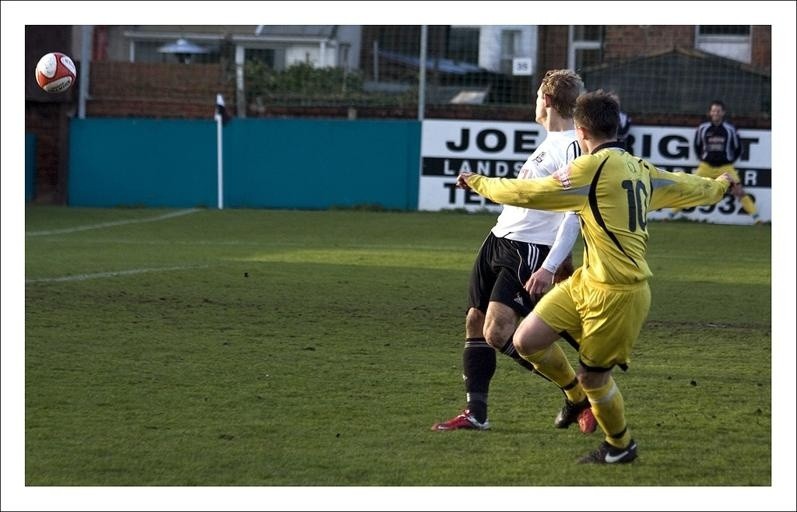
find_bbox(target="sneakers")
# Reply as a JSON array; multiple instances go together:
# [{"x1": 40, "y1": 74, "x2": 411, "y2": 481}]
[
  {"x1": 577, "y1": 441, "x2": 637, "y2": 465},
  {"x1": 432, "y1": 409, "x2": 490, "y2": 431},
  {"x1": 555, "y1": 394, "x2": 598, "y2": 433}
]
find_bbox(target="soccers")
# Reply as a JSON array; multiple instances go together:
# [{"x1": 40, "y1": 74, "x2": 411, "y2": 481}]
[{"x1": 35, "y1": 52, "x2": 76, "y2": 93}]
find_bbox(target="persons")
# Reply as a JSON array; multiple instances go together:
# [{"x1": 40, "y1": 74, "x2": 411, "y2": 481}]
[
  {"x1": 424, "y1": 67, "x2": 598, "y2": 437},
  {"x1": 451, "y1": 88, "x2": 739, "y2": 467},
  {"x1": 661, "y1": 99, "x2": 763, "y2": 227}
]
